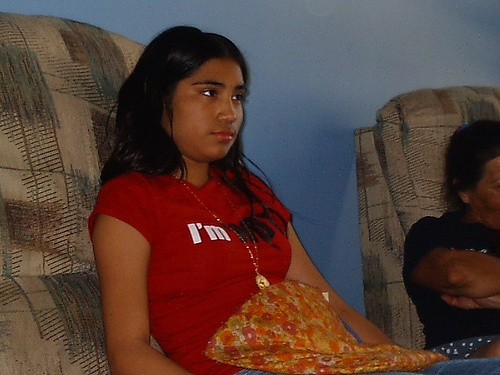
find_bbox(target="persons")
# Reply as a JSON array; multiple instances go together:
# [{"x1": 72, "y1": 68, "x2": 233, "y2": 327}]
[
  {"x1": 401, "y1": 119, "x2": 500, "y2": 360},
  {"x1": 86, "y1": 26, "x2": 500, "y2": 375}
]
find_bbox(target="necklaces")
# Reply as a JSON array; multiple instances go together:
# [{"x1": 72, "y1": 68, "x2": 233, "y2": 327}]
[{"x1": 173, "y1": 169, "x2": 272, "y2": 290}]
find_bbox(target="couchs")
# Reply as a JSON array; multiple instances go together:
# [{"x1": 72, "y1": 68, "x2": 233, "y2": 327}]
[
  {"x1": 0, "y1": 12, "x2": 147, "y2": 375},
  {"x1": 354, "y1": 86, "x2": 500, "y2": 348}
]
